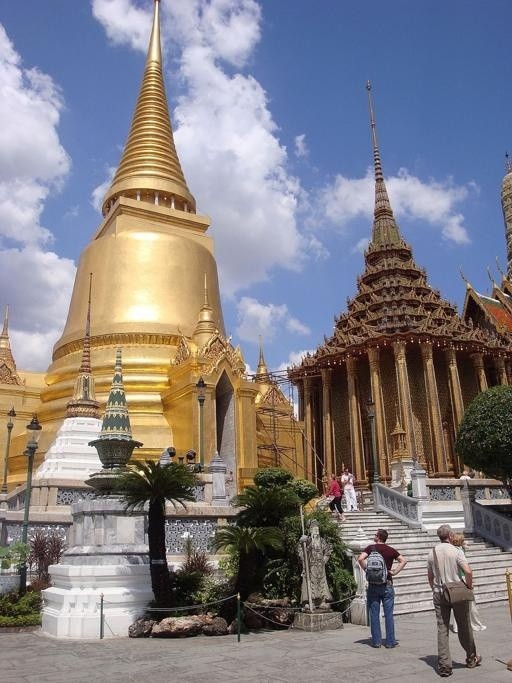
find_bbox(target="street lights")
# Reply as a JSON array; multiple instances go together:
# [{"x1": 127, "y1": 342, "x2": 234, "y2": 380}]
[
  {"x1": 17, "y1": 415, "x2": 43, "y2": 601},
  {"x1": 4, "y1": 405, "x2": 14, "y2": 492}
]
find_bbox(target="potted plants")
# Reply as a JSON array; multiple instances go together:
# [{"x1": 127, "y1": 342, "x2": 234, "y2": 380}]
[{"x1": 0, "y1": 540, "x2": 31, "y2": 595}]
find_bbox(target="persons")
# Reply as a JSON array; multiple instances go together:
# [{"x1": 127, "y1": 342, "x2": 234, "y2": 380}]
[
  {"x1": 449, "y1": 531, "x2": 487, "y2": 634},
  {"x1": 325, "y1": 472, "x2": 347, "y2": 523},
  {"x1": 357, "y1": 529, "x2": 408, "y2": 649},
  {"x1": 426, "y1": 523, "x2": 483, "y2": 678},
  {"x1": 340, "y1": 468, "x2": 360, "y2": 512}
]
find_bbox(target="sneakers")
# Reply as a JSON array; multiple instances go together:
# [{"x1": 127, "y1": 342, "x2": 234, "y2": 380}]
[
  {"x1": 439, "y1": 667, "x2": 453, "y2": 677},
  {"x1": 473, "y1": 625, "x2": 487, "y2": 631},
  {"x1": 467, "y1": 654, "x2": 481, "y2": 668},
  {"x1": 453, "y1": 630, "x2": 458, "y2": 632},
  {"x1": 370, "y1": 640, "x2": 400, "y2": 648}
]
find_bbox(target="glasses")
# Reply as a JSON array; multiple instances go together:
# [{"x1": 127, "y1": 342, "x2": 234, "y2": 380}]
[{"x1": 375, "y1": 534, "x2": 377, "y2": 537}]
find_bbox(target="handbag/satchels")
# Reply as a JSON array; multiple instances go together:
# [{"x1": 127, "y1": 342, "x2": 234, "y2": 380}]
[
  {"x1": 365, "y1": 552, "x2": 388, "y2": 585},
  {"x1": 443, "y1": 581, "x2": 475, "y2": 604}
]
